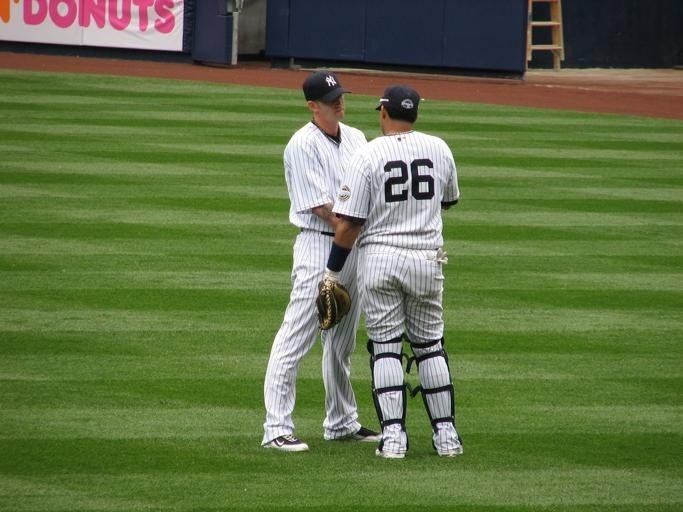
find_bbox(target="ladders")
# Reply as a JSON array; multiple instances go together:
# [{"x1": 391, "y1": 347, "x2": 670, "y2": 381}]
[{"x1": 525, "y1": 0, "x2": 565, "y2": 72}]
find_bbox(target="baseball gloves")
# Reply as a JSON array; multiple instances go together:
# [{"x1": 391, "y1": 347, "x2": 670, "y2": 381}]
[{"x1": 316, "y1": 279, "x2": 351, "y2": 329}]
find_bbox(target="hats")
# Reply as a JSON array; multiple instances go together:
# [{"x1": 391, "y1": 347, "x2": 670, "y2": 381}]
[
  {"x1": 375, "y1": 86, "x2": 420, "y2": 110},
  {"x1": 303, "y1": 69, "x2": 352, "y2": 103}
]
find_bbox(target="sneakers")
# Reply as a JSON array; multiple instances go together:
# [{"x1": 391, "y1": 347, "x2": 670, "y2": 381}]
[
  {"x1": 269, "y1": 433, "x2": 309, "y2": 451},
  {"x1": 375, "y1": 446, "x2": 405, "y2": 459},
  {"x1": 350, "y1": 427, "x2": 384, "y2": 441}
]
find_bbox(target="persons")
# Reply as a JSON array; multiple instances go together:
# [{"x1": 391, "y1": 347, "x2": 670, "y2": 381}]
[
  {"x1": 322, "y1": 84, "x2": 463, "y2": 460},
  {"x1": 259, "y1": 70, "x2": 386, "y2": 454}
]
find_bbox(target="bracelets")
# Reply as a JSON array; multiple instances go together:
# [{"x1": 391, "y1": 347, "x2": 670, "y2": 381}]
[{"x1": 326, "y1": 241, "x2": 351, "y2": 272}]
